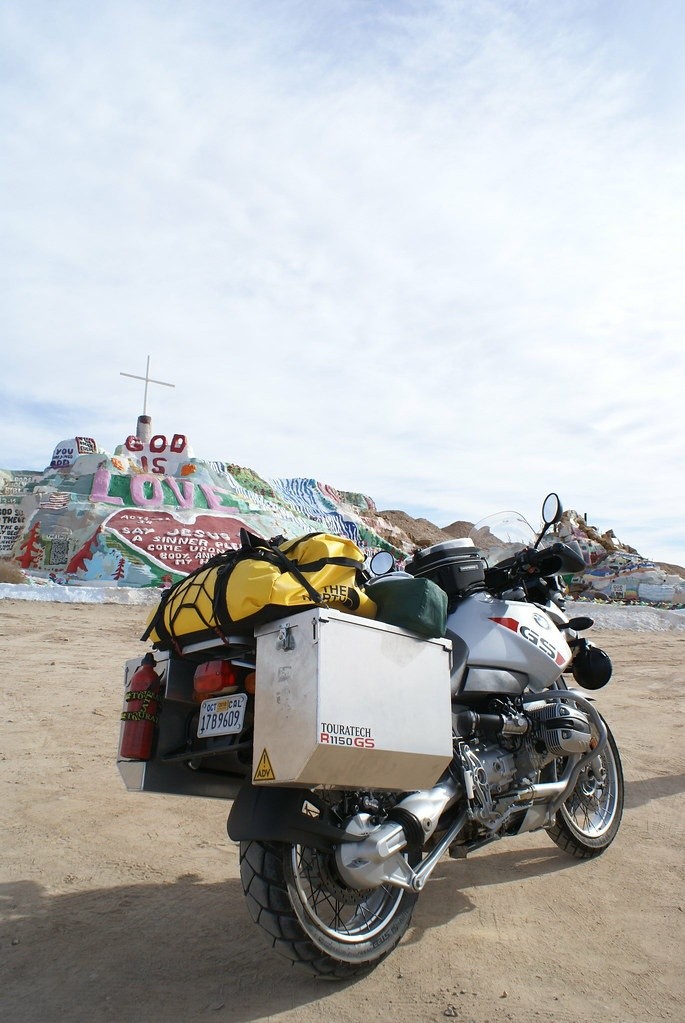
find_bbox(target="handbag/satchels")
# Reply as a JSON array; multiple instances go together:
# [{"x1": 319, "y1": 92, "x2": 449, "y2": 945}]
[
  {"x1": 140, "y1": 529, "x2": 375, "y2": 650},
  {"x1": 368, "y1": 572, "x2": 449, "y2": 638}
]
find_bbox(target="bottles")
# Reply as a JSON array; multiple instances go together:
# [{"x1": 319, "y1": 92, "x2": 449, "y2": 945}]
[{"x1": 121, "y1": 652, "x2": 160, "y2": 759}]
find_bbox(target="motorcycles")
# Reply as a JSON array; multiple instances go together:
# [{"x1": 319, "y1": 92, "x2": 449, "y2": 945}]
[{"x1": 114, "y1": 492, "x2": 625, "y2": 985}]
